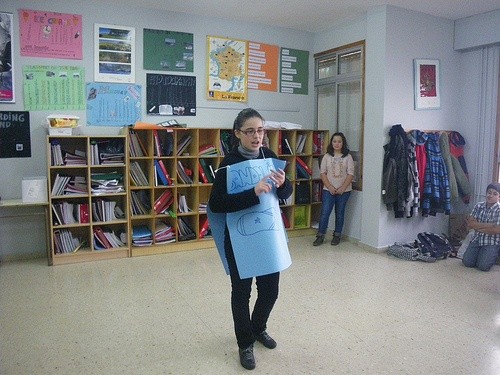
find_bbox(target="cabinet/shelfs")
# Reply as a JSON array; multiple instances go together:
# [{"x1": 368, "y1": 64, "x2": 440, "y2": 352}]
[{"x1": 46, "y1": 127, "x2": 330, "y2": 265}]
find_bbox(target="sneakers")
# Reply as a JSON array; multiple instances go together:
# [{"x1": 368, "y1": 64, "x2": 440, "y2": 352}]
[
  {"x1": 259, "y1": 332, "x2": 277, "y2": 349},
  {"x1": 239, "y1": 345, "x2": 256, "y2": 370}
]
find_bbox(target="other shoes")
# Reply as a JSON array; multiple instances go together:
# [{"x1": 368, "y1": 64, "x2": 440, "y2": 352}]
[
  {"x1": 313, "y1": 237, "x2": 324, "y2": 246},
  {"x1": 331, "y1": 237, "x2": 340, "y2": 246}
]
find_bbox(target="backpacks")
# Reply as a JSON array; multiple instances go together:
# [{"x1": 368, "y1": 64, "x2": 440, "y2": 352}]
[{"x1": 417, "y1": 232, "x2": 448, "y2": 258}]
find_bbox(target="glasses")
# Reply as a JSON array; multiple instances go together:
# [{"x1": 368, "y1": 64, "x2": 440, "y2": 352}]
[{"x1": 240, "y1": 129, "x2": 264, "y2": 135}]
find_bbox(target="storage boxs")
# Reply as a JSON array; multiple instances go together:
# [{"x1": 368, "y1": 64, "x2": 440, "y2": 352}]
[{"x1": 21, "y1": 175, "x2": 47, "y2": 202}]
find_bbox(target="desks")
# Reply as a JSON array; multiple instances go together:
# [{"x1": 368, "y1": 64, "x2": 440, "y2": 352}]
[{"x1": 0, "y1": 198, "x2": 51, "y2": 267}]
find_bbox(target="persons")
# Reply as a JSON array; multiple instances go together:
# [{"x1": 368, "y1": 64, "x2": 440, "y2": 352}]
[
  {"x1": 313, "y1": 132, "x2": 354, "y2": 246},
  {"x1": 207, "y1": 109, "x2": 293, "y2": 369},
  {"x1": 462, "y1": 183, "x2": 500, "y2": 271}
]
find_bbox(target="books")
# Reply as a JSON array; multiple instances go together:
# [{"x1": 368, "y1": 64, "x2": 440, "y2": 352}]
[
  {"x1": 153, "y1": 130, "x2": 229, "y2": 157},
  {"x1": 54, "y1": 228, "x2": 81, "y2": 254},
  {"x1": 130, "y1": 160, "x2": 149, "y2": 186},
  {"x1": 90, "y1": 140, "x2": 124, "y2": 165},
  {"x1": 49, "y1": 142, "x2": 87, "y2": 166},
  {"x1": 92, "y1": 198, "x2": 123, "y2": 222},
  {"x1": 279, "y1": 131, "x2": 323, "y2": 179},
  {"x1": 132, "y1": 225, "x2": 153, "y2": 246},
  {"x1": 94, "y1": 226, "x2": 126, "y2": 249},
  {"x1": 130, "y1": 191, "x2": 150, "y2": 215},
  {"x1": 91, "y1": 171, "x2": 125, "y2": 194},
  {"x1": 313, "y1": 182, "x2": 322, "y2": 201},
  {"x1": 52, "y1": 172, "x2": 89, "y2": 196},
  {"x1": 154, "y1": 159, "x2": 215, "y2": 187},
  {"x1": 52, "y1": 200, "x2": 89, "y2": 225},
  {"x1": 155, "y1": 189, "x2": 210, "y2": 245},
  {"x1": 130, "y1": 130, "x2": 148, "y2": 157}
]
